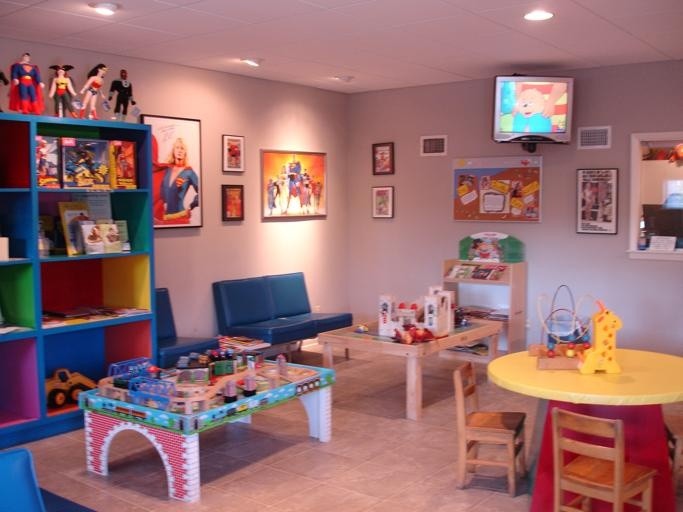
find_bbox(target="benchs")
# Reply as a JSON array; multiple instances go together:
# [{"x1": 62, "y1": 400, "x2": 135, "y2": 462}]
[{"x1": 211, "y1": 272, "x2": 353, "y2": 362}]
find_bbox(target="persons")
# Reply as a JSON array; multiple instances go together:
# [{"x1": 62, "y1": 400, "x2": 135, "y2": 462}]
[
  {"x1": 300, "y1": 168, "x2": 324, "y2": 214},
  {"x1": 159, "y1": 138, "x2": 199, "y2": 224},
  {"x1": 50, "y1": 65, "x2": 76, "y2": 117},
  {"x1": 80, "y1": 64, "x2": 106, "y2": 117},
  {"x1": 267, "y1": 178, "x2": 277, "y2": 215},
  {"x1": 272, "y1": 174, "x2": 287, "y2": 214},
  {"x1": 7, "y1": 53, "x2": 46, "y2": 116},
  {"x1": 283, "y1": 153, "x2": 306, "y2": 215},
  {"x1": 107, "y1": 69, "x2": 136, "y2": 115}
]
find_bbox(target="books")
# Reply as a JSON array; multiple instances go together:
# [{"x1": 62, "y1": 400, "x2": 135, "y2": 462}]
[
  {"x1": 213, "y1": 336, "x2": 272, "y2": 354},
  {"x1": 448, "y1": 264, "x2": 507, "y2": 280},
  {"x1": 39, "y1": 306, "x2": 142, "y2": 326},
  {"x1": 456, "y1": 305, "x2": 509, "y2": 321}
]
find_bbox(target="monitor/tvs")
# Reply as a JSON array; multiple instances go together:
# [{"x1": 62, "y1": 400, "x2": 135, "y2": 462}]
[{"x1": 490, "y1": 75, "x2": 574, "y2": 153}]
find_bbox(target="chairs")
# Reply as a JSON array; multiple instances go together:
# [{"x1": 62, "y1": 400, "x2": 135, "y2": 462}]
[
  {"x1": 552, "y1": 408, "x2": 658, "y2": 512},
  {"x1": 156, "y1": 288, "x2": 218, "y2": 368},
  {"x1": 455, "y1": 359, "x2": 527, "y2": 496}
]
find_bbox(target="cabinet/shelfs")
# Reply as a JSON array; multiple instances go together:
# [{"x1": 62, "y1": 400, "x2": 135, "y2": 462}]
[
  {"x1": 0, "y1": 111, "x2": 157, "y2": 450},
  {"x1": 442, "y1": 260, "x2": 527, "y2": 362},
  {"x1": 629, "y1": 132, "x2": 683, "y2": 258}
]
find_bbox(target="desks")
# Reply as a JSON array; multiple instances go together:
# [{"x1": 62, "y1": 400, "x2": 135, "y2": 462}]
[
  {"x1": 78, "y1": 355, "x2": 336, "y2": 501},
  {"x1": 487, "y1": 347, "x2": 682, "y2": 480},
  {"x1": 318, "y1": 317, "x2": 503, "y2": 421}
]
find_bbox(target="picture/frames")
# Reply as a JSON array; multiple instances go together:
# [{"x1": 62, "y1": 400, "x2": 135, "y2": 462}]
[
  {"x1": 371, "y1": 142, "x2": 394, "y2": 175},
  {"x1": 222, "y1": 134, "x2": 245, "y2": 172},
  {"x1": 260, "y1": 150, "x2": 328, "y2": 222},
  {"x1": 140, "y1": 114, "x2": 203, "y2": 229},
  {"x1": 372, "y1": 186, "x2": 394, "y2": 218},
  {"x1": 221, "y1": 185, "x2": 244, "y2": 221},
  {"x1": 577, "y1": 168, "x2": 618, "y2": 235}
]
